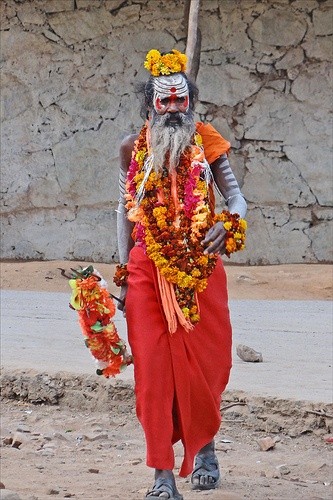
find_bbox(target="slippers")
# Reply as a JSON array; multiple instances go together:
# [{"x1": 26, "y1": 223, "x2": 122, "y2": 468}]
[
  {"x1": 190, "y1": 454, "x2": 221, "y2": 490},
  {"x1": 144, "y1": 476, "x2": 183, "y2": 500}
]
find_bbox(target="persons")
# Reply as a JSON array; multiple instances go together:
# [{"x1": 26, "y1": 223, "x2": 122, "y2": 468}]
[{"x1": 113, "y1": 50, "x2": 249, "y2": 500}]
